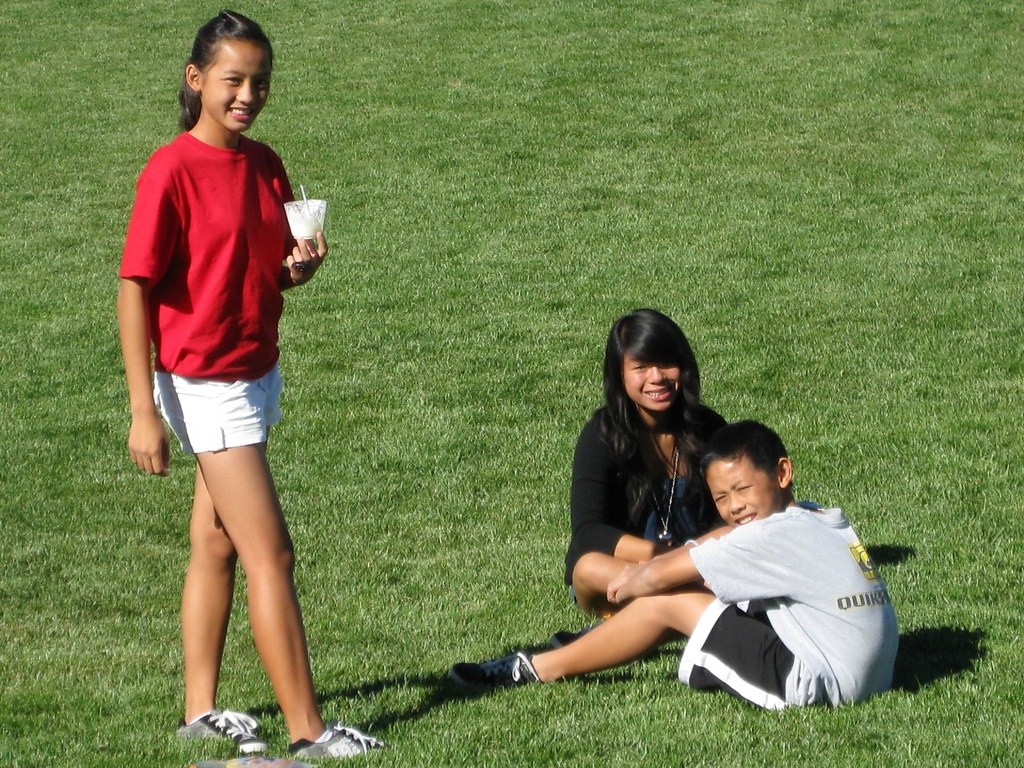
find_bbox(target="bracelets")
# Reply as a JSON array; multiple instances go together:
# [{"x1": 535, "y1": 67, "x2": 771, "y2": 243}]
[{"x1": 684, "y1": 540, "x2": 698, "y2": 546}]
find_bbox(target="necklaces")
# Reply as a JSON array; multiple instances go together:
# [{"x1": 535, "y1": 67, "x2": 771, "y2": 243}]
[{"x1": 649, "y1": 450, "x2": 679, "y2": 542}]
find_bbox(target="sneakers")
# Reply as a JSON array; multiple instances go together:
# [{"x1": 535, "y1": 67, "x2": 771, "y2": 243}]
[
  {"x1": 549, "y1": 628, "x2": 589, "y2": 649},
  {"x1": 288, "y1": 720, "x2": 385, "y2": 759},
  {"x1": 173, "y1": 710, "x2": 268, "y2": 753},
  {"x1": 450, "y1": 648, "x2": 566, "y2": 692}
]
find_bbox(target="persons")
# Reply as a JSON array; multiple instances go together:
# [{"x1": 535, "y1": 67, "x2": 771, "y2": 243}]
[
  {"x1": 562, "y1": 308, "x2": 729, "y2": 619},
  {"x1": 117, "y1": 10, "x2": 383, "y2": 760},
  {"x1": 449, "y1": 420, "x2": 899, "y2": 712}
]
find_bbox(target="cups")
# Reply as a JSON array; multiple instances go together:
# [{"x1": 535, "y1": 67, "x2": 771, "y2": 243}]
[{"x1": 284, "y1": 200, "x2": 327, "y2": 240}]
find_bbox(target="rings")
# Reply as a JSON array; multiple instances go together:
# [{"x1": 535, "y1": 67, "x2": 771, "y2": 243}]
[{"x1": 291, "y1": 262, "x2": 306, "y2": 272}]
